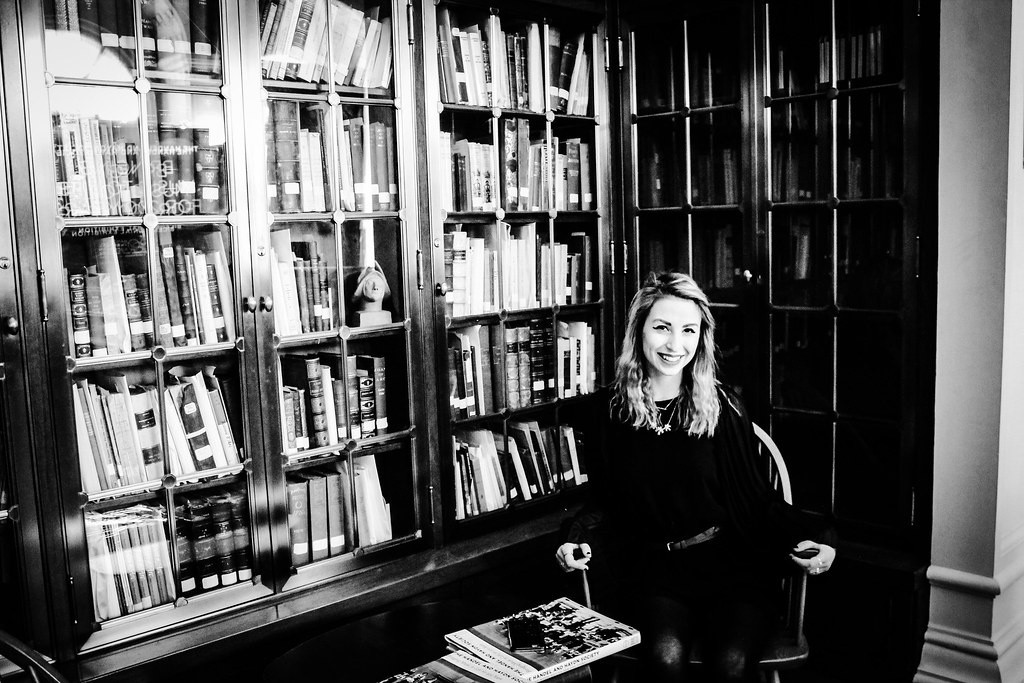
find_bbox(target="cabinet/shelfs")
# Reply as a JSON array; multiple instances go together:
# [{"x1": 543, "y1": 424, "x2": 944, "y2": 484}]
[{"x1": 0, "y1": 0, "x2": 942, "y2": 683}]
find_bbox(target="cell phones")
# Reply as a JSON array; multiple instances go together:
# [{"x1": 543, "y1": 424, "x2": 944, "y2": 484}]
[{"x1": 506, "y1": 619, "x2": 545, "y2": 652}]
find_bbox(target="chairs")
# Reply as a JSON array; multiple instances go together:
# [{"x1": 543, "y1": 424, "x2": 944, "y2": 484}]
[{"x1": 563, "y1": 419, "x2": 819, "y2": 683}]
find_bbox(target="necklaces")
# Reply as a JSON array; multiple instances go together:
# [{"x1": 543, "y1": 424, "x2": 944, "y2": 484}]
[
  {"x1": 655, "y1": 395, "x2": 678, "y2": 410},
  {"x1": 656, "y1": 404, "x2": 677, "y2": 435}
]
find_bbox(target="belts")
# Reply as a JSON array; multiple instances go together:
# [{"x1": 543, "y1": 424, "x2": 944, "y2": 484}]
[{"x1": 666, "y1": 526, "x2": 720, "y2": 552}]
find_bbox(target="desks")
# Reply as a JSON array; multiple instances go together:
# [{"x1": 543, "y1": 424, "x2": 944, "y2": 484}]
[{"x1": 244, "y1": 601, "x2": 592, "y2": 683}]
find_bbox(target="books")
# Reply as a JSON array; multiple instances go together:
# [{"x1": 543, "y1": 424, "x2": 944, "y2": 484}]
[
  {"x1": 444, "y1": 597, "x2": 642, "y2": 683},
  {"x1": 40, "y1": 1, "x2": 906, "y2": 626},
  {"x1": 378, "y1": 650, "x2": 530, "y2": 683}
]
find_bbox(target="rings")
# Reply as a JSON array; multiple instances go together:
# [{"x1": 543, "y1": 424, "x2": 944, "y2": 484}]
[
  {"x1": 817, "y1": 567, "x2": 820, "y2": 574},
  {"x1": 559, "y1": 562, "x2": 566, "y2": 566},
  {"x1": 817, "y1": 558, "x2": 823, "y2": 565},
  {"x1": 563, "y1": 552, "x2": 572, "y2": 557}
]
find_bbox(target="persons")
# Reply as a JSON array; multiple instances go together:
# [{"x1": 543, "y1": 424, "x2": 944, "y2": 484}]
[
  {"x1": 555, "y1": 273, "x2": 839, "y2": 683},
  {"x1": 381, "y1": 602, "x2": 625, "y2": 683}
]
